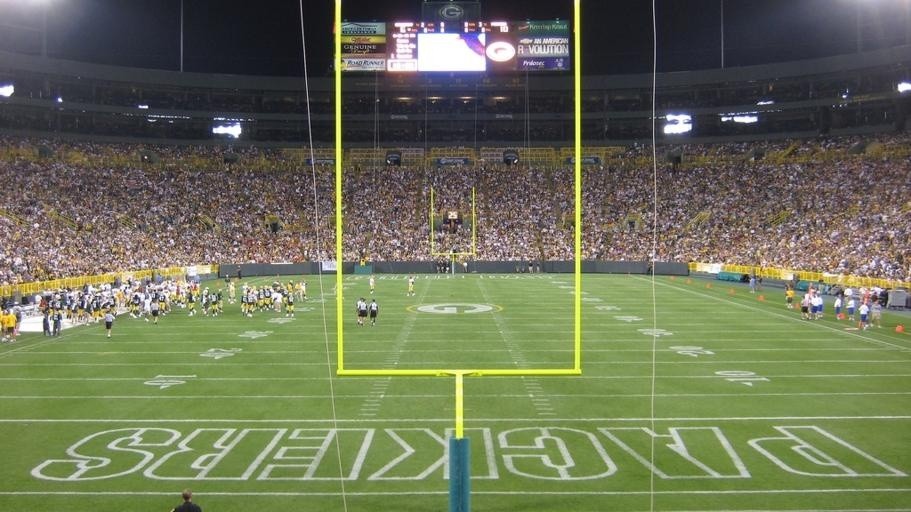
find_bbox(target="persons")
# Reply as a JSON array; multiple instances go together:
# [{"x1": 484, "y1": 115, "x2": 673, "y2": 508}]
[
  {"x1": 369, "y1": 274, "x2": 375, "y2": 294},
  {"x1": 0, "y1": 132, "x2": 911, "y2": 283},
  {"x1": 356, "y1": 298, "x2": 378, "y2": 326},
  {"x1": 407, "y1": 276, "x2": 415, "y2": 296},
  {"x1": 785, "y1": 280, "x2": 883, "y2": 330},
  {"x1": 170, "y1": 488, "x2": 202, "y2": 512},
  {"x1": 29, "y1": 274, "x2": 236, "y2": 338},
  {"x1": 750, "y1": 274, "x2": 762, "y2": 293},
  {"x1": 241, "y1": 279, "x2": 308, "y2": 318},
  {"x1": 0, "y1": 298, "x2": 22, "y2": 342}
]
